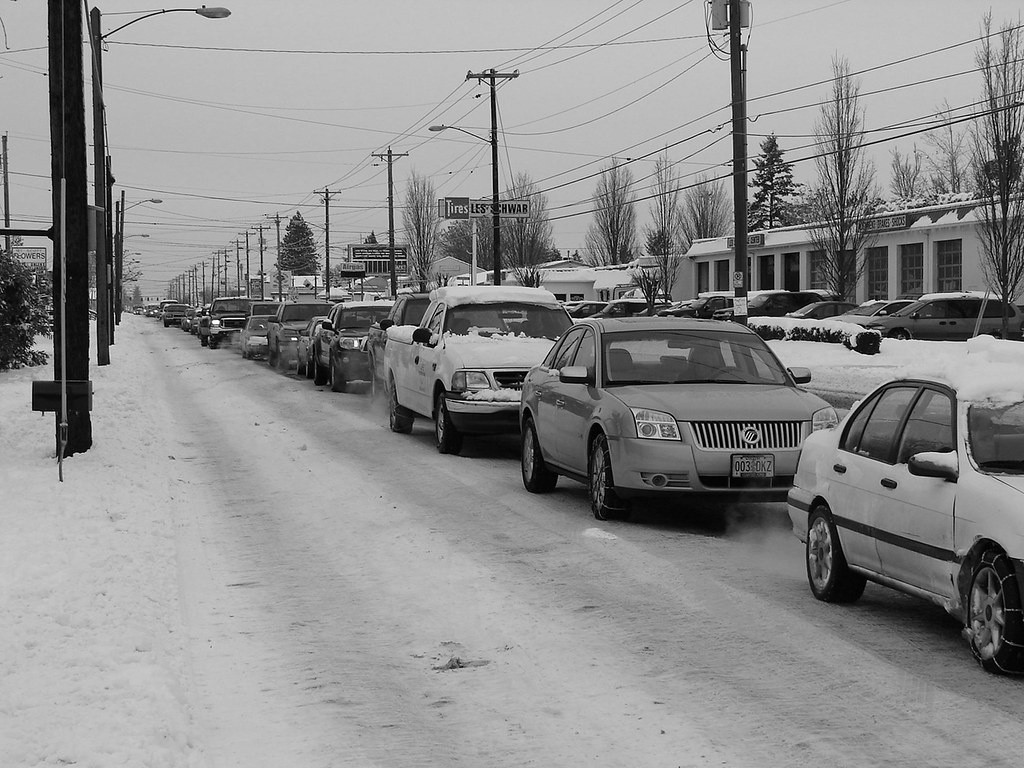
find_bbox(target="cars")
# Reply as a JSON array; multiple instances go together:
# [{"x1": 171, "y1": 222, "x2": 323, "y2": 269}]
[
  {"x1": 128, "y1": 276, "x2": 1024, "y2": 398},
  {"x1": 517, "y1": 314, "x2": 840, "y2": 523},
  {"x1": 785, "y1": 368, "x2": 1024, "y2": 681}
]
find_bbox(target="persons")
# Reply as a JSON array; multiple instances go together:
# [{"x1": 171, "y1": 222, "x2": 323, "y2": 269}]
[{"x1": 679, "y1": 346, "x2": 723, "y2": 382}]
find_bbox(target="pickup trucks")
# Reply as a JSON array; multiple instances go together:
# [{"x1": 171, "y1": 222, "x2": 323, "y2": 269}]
[
  {"x1": 366, "y1": 289, "x2": 510, "y2": 415},
  {"x1": 383, "y1": 284, "x2": 584, "y2": 454}
]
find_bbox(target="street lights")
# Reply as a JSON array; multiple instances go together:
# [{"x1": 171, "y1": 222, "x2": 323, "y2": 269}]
[
  {"x1": 114, "y1": 198, "x2": 164, "y2": 325},
  {"x1": 89, "y1": 5, "x2": 231, "y2": 368},
  {"x1": 430, "y1": 124, "x2": 502, "y2": 285}
]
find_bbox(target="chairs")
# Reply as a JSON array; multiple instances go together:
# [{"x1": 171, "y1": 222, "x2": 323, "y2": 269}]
[
  {"x1": 609, "y1": 348, "x2": 633, "y2": 380},
  {"x1": 376, "y1": 315, "x2": 386, "y2": 323},
  {"x1": 345, "y1": 316, "x2": 358, "y2": 327},
  {"x1": 994, "y1": 434, "x2": 1024, "y2": 461},
  {"x1": 660, "y1": 346, "x2": 721, "y2": 382},
  {"x1": 446, "y1": 318, "x2": 471, "y2": 335},
  {"x1": 219, "y1": 306, "x2": 225, "y2": 310}
]
